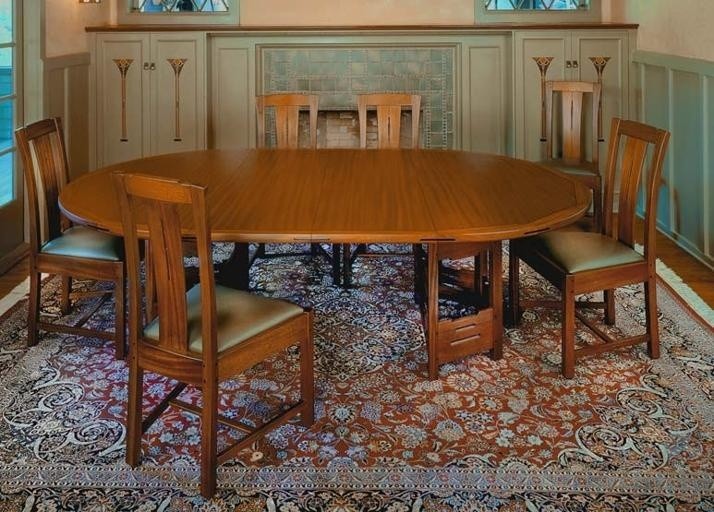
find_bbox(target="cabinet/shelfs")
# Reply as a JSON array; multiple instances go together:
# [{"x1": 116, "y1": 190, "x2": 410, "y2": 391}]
[
  {"x1": 90, "y1": 32, "x2": 210, "y2": 174},
  {"x1": 508, "y1": 29, "x2": 641, "y2": 218}
]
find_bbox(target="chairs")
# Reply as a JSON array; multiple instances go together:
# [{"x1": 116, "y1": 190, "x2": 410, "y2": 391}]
[
  {"x1": 344, "y1": 91, "x2": 423, "y2": 286},
  {"x1": 510, "y1": 116, "x2": 672, "y2": 376},
  {"x1": 114, "y1": 170, "x2": 317, "y2": 495},
  {"x1": 15, "y1": 116, "x2": 149, "y2": 361},
  {"x1": 246, "y1": 93, "x2": 342, "y2": 284},
  {"x1": 549, "y1": 83, "x2": 607, "y2": 240}
]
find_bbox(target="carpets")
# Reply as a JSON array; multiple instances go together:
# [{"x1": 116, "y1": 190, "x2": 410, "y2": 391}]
[{"x1": 0, "y1": 241, "x2": 714, "y2": 512}]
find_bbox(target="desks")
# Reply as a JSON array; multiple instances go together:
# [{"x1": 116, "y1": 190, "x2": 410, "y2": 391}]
[{"x1": 56, "y1": 145, "x2": 592, "y2": 382}]
